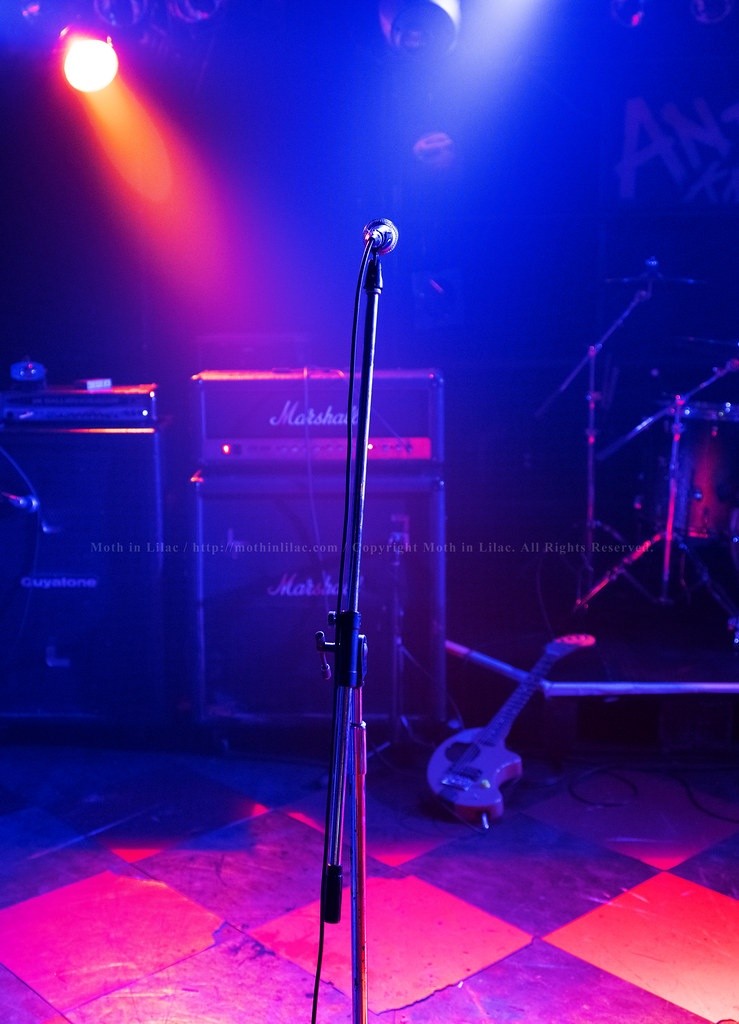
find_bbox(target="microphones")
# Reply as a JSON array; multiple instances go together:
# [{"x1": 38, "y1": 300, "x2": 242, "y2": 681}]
[{"x1": 363, "y1": 217, "x2": 399, "y2": 255}]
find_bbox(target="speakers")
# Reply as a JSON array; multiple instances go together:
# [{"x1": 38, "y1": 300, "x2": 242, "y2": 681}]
[
  {"x1": 189, "y1": 474, "x2": 449, "y2": 728},
  {"x1": 0, "y1": 427, "x2": 175, "y2": 733}
]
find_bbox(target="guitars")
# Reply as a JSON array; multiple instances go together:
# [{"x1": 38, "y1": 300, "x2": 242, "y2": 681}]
[{"x1": 424, "y1": 633, "x2": 597, "y2": 824}]
